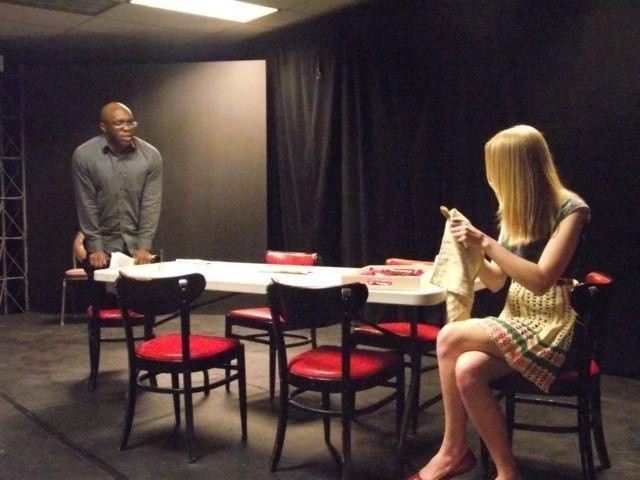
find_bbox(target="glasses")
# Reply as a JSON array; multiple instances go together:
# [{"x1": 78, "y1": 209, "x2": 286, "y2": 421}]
[{"x1": 104, "y1": 119, "x2": 139, "y2": 130}]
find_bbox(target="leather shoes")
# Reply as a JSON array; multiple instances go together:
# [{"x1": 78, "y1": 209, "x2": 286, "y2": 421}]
[{"x1": 407, "y1": 448, "x2": 477, "y2": 480}]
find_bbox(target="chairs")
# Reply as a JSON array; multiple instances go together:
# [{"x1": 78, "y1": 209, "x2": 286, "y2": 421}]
[
  {"x1": 58, "y1": 245, "x2": 91, "y2": 325},
  {"x1": 348, "y1": 258, "x2": 464, "y2": 434},
  {"x1": 266, "y1": 279, "x2": 407, "y2": 474},
  {"x1": 88, "y1": 256, "x2": 164, "y2": 391},
  {"x1": 479, "y1": 271, "x2": 614, "y2": 480},
  {"x1": 116, "y1": 271, "x2": 249, "y2": 462},
  {"x1": 224, "y1": 247, "x2": 319, "y2": 394}
]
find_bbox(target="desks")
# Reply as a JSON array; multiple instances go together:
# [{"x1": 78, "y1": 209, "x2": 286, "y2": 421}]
[{"x1": 91, "y1": 258, "x2": 487, "y2": 447}]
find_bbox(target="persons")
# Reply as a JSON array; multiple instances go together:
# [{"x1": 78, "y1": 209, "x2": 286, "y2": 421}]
[
  {"x1": 70, "y1": 101, "x2": 164, "y2": 311},
  {"x1": 407, "y1": 123, "x2": 592, "y2": 479}
]
